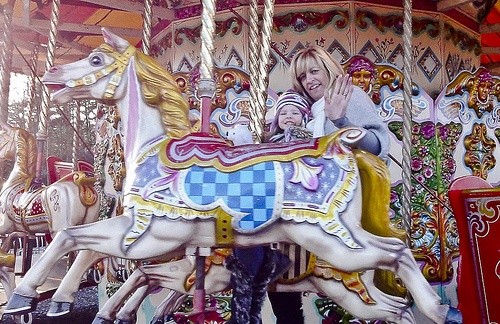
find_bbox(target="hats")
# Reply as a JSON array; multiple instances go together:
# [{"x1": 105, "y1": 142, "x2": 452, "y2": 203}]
[{"x1": 269, "y1": 89, "x2": 313, "y2": 133}]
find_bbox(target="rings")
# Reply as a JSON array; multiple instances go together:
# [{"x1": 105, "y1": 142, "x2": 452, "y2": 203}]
[{"x1": 343, "y1": 93, "x2": 347, "y2": 96}]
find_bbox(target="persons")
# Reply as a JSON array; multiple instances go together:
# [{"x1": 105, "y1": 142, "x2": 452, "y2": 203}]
[
  {"x1": 264, "y1": 88, "x2": 314, "y2": 144},
  {"x1": 288, "y1": 44, "x2": 390, "y2": 161}
]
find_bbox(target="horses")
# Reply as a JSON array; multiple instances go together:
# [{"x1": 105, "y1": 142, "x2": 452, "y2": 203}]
[
  {"x1": 5, "y1": 26, "x2": 463, "y2": 324},
  {"x1": 0, "y1": 119, "x2": 100, "y2": 257},
  {"x1": 92, "y1": 242, "x2": 415, "y2": 324}
]
